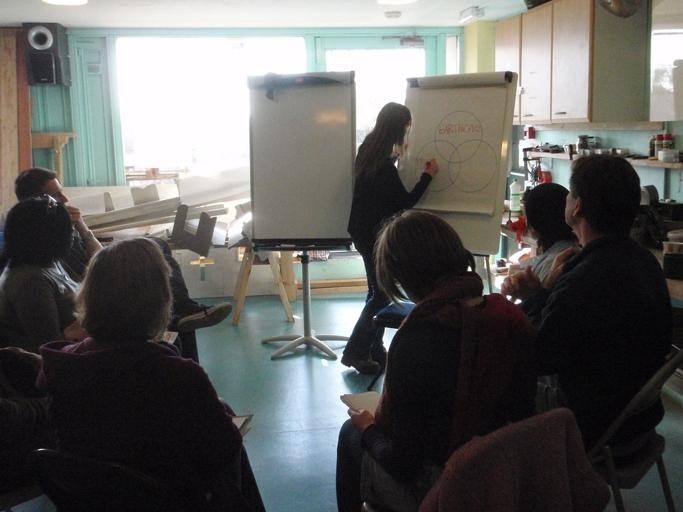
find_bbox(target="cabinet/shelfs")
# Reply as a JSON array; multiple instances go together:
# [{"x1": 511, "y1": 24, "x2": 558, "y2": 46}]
[
  {"x1": 492, "y1": 4, "x2": 554, "y2": 124},
  {"x1": 548, "y1": 1, "x2": 649, "y2": 128}
]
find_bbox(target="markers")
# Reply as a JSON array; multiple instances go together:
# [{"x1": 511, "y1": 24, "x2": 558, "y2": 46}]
[{"x1": 280, "y1": 244, "x2": 297, "y2": 248}]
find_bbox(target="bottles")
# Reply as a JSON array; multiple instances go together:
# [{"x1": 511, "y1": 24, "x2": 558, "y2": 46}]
[
  {"x1": 648, "y1": 133, "x2": 657, "y2": 158},
  {"x1": 103, "y1": 191, "x2": 115, "y2": 213},
  {"x1": 653, "y1": 131, "x2": 672, "y2": 158},
  {"x1": 508, "y1": 176, "x2": 522, "y2": 214}
]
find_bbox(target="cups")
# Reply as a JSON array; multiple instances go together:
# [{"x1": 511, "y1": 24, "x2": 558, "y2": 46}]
[{"x1": 496, "y1": 258, "x2": 507, "y2": 268}]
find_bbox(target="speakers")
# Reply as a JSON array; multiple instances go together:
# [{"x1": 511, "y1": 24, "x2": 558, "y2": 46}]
[{"x1": 23, "y1": 23, "x2": 71, "y2": 87}]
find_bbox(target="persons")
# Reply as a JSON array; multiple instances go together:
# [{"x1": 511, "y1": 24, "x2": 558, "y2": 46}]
[
  {"x1": 1, "y1": 346, "x2": 55, "y2": 482},
  {"x1": 340, "y1": 102, "x2": 439, "y2": 375},
  {"x1": 507, "y1": 184, "x2": 579, "y2": 306},
  {"x1": 336, "y1": 211, "x2": 541, "y2": 509},
  {"x1": 502, "y1": 152, "x2": 666, "y2": 459},
  {"x1": 34, "y1": 235, "x2": 264, "y2": 511},
  {"x1": 15, "y1": 170, "x2": 233, "y2": 365},
  {"x1": 2, "y1": 194, "x2": 237, "y2": 416}
]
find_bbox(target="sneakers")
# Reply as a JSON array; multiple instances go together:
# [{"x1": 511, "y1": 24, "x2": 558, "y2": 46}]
[
  {"x1": 176, "y1": 300, "x2": 232, "y2": 333},
  {"x1": 341, "y1": 354, "x2": 382, "y2": 374}
]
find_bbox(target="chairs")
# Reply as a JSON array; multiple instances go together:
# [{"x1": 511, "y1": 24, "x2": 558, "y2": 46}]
[{"x1": 588, "y1": 352, "x2": 682, "y2": 512}]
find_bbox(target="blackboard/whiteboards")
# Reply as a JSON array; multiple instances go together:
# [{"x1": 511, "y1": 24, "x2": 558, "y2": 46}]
[
  {"x1": 398, "y1": 72, "x2": 517, "y2": 256},
  {"x1": 249, "y1": 70, "x2": 355, "y2": 251}
]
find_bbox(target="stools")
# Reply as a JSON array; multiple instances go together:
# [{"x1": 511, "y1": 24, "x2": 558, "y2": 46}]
[{"x1": 364, "y1": 300, "x2": 416, "y2": 393}]
